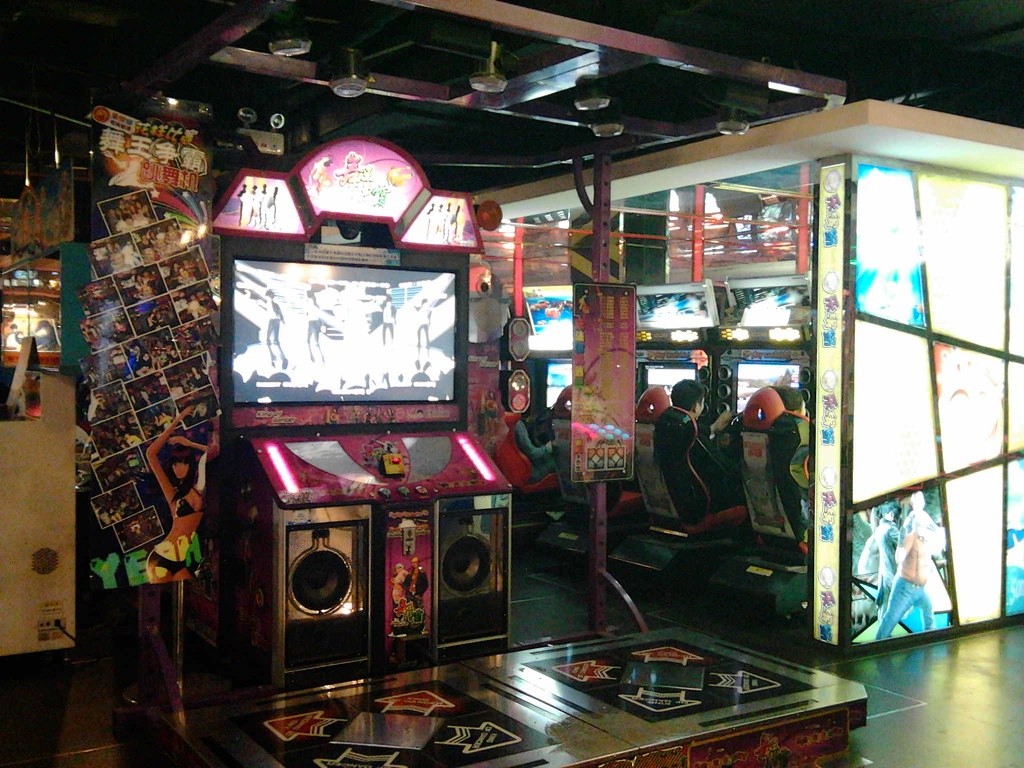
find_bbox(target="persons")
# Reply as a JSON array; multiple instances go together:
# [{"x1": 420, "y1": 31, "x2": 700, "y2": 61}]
[
  {"x1": 79, "y1": 186, "x2": 224, "y2": 555},
  {"x1": 664, "y1": 379, "x2": 746, "y2": 497},
  {"x1": 514, "y1": 404, "x2": 559, "y2": 483},
  {"x1": 767, "y1": 384, "x2": 810, "y2": 420},
  {"x1": 857, "y1": 490, "x2": 947, "y2": 641}
]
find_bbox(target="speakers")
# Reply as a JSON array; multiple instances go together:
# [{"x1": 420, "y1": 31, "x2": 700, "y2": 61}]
[
  {"x1": 433, "y1": 492, "x2": 511, "y2": 662},
  {"x1": 274, "y1": 504, "x2": 373, "y2": 688}
]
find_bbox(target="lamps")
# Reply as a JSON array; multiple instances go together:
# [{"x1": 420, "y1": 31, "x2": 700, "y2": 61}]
[
  {"x1": 468, "y1": 39, "x2": 507, "y2": 94},
  {"x1": 332, "y1": 46, "x2": 369, "y2": 98},
  {"x1": 573, "y1": 84, "x2": 611, "y2": 110},
  {"x1": 268, "y1": 4, "x2": 311, "y2": 56},
  {"x1": 235, "y1": 107, "x2": 285, "y2": 156},
  {"x1": 590, "y1": 105, "x2": 624, "y2": 137},
  {"x1": 716, "y1": 103, "x2": 750, "y2": 135}
]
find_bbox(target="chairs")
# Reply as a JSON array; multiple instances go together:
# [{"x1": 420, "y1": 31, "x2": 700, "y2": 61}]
[{"x1": 493, "y1": 379, "x2": 810, "y2": 560}]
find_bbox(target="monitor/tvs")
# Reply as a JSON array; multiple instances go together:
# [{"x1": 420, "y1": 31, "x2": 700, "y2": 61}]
[
  {"x1": 543, "y1": 360, "x2": 816, "y2": 418},
  {"x1": 219, "y1": 235, "x2": 470, "y2": 439}
]
